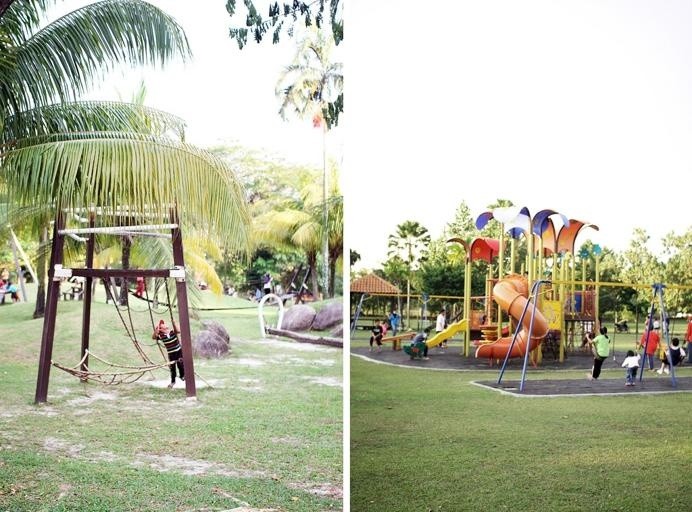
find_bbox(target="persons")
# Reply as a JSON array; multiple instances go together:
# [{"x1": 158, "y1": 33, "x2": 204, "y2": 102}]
[
  {"x1": 228, "y1": 273, "x2": 272, "y2": 304},
  {"x1": 619, "y1": 318, "x2": 630, "y2": 334},
  {"x1": 585, "y1": 326, "x2": 610, "y2": 383},
  {"x1": 566, "y1": 328, "x2": 577, "y2": 348},
  {"x1": 636, "y1": 314, "x2": 692, "y2": 374},
  {"x1": 621, "y1": 350, "x2": 641, "y2": 386},
  {"x1": 369, "y1": 309, "x2": 449, "y2": 361},
  {"x1": 135, "y1": 267, "x2": 145, "y2": 297},
  {"x1": 0, "y1": 269, "x2": 19, "y2": 304},
  {"x1": 151, "y1": 318, "x2": 186, "y2": 389}
]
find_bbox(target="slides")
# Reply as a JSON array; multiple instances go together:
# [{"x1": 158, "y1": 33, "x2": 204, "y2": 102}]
[
  {"x1": 476, "y1": 273, "x2": 549, "y2": 359},
  {"x1": 420, "y1": 317, "x2": 465, "y2": 351}
]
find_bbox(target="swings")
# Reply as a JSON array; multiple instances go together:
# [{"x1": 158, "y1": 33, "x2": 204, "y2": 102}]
[
  {"x1": 661, "y1": 287, "x2": 687, "y2": 365},
  {"x1": 101, "y1": 270, "x2": 185, "y2": 379},
  {"x1": 612, "y1": 288, "x2": 641, "y2": 367},
  {"x1": 568, "y1": 290, "x2": 610, "y2": 361}
]
